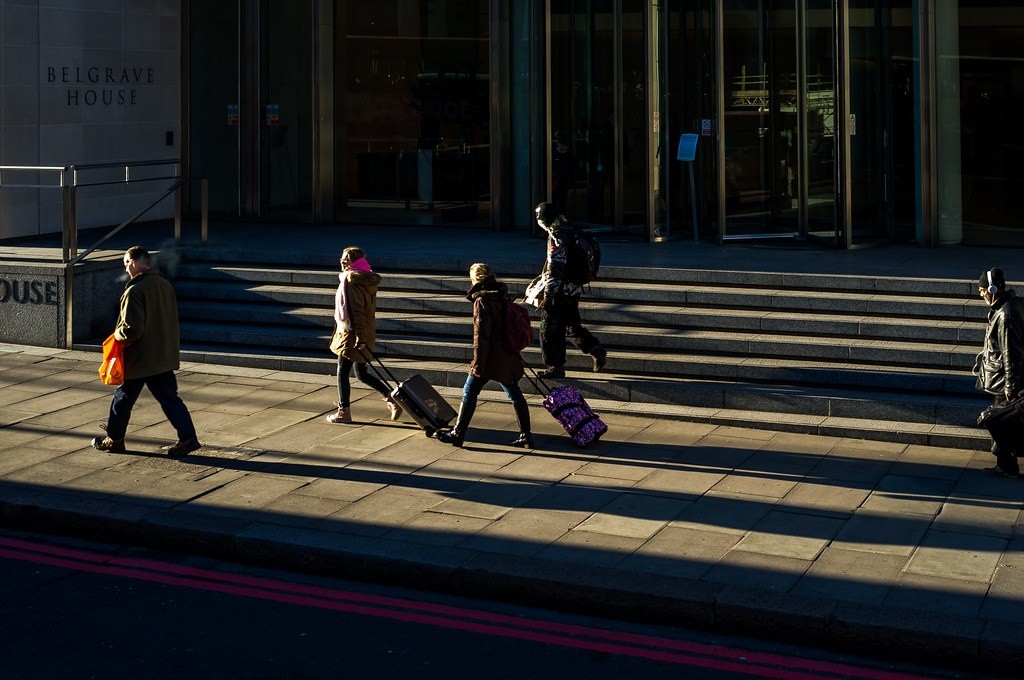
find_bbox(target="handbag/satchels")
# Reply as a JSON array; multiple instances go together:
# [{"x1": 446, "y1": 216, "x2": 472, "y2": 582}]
[
  {"x1": 97, "y1": 334, "x2": 125, "y2": 385},
  {"x1": 522, "y1": 275, "x2": 556, "y2": 308},
  {"x1": 543, "y1": 278, "x2": 582, "y2": 317}
]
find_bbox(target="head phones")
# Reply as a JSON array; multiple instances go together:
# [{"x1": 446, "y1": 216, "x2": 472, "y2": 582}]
[{"x1": 987, "y1": 271, "x2": 998, "y2": 294}]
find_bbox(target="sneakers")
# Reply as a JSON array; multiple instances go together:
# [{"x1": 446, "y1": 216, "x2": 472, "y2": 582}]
[
  {"x1": 592, "y1": 348, "x2": 608, "y2": 372},
  {"x1": 167, "y1": 436, "x2": 202, "y2": 457},
  {"x1": 983, "y1": 466, "x2": 1021, "y2": 478},
  {"x1": 538, "y1": 359, "x2": 566, "y2": 379},
  {"x1": 90, "y1": 423, "x2": 126, "y2": 454}
]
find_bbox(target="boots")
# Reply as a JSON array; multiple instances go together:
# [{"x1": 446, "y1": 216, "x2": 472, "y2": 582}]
[
  {"x1": 326, "y1": 407, "x2": 352, "y2": 423},
  {"x1": 382, "y1": 396, "x2": 403, "y2": 421},
  {"x1": 511, "y1": 403, "x2": 534, "y2": 448},
  {"x1": 435, "y1": 401, "x2": 478, "y2": 447}
]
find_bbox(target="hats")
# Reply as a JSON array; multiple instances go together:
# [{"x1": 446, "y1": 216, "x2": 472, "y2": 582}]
[{"x1": 977, "y1": 267, "x2": 1005, "y2": 288}]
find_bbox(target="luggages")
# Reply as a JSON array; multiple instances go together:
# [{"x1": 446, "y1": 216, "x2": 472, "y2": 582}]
[
  {"x1": 521, "y1": 360, "x2": 608, "y2": 448},
  {"x1": 357, "y1": 341, "x2": 459, "y2": 438}
]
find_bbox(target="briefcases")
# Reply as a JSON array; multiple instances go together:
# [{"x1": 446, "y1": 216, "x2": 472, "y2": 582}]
[{"x1": 976, "y1": 390, "x2": 1024, "y2": 458}]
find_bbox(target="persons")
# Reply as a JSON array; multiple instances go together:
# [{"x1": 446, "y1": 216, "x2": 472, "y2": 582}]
[
  {"x1": 532, "y1": 201, "x2": 608, "y2": 380},
  {"x1": 325, "y1": 247, "x2": 403, "y2": 423},
  {"x1": 435, "y1": 263, "x2": 536, "y2": 449},
  {"x1": 972, "y1": 265, "x2": 1024, "y2": 480},
  {"x1": 90, "y1": 245, "x2": 202, "y2": 458}
]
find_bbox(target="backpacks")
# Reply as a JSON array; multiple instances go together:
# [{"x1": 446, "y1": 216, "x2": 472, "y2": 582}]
[
  {"x1": 551, "y1": 214, "x2": 601, "y2": 287},
  {"x1": 479, "y1": 293, "x2": 534, "y2": 354}
]
